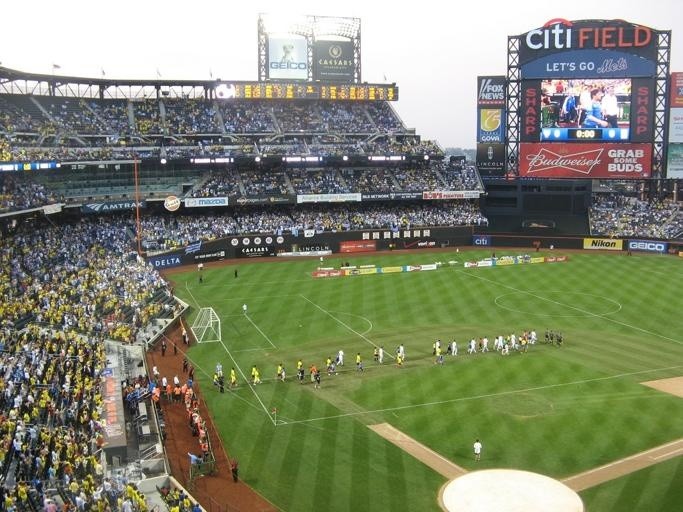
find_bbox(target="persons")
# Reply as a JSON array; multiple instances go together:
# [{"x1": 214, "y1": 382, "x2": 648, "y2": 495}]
[
  {"x1": 538, "y1": 80, "x2": 633, "y2": 140},
  {"x1": 547, "y1": 329, "x2": 554, "y2": 344},
  {"x1": 592, "y1": 187, "x2": 681, "y2": 237},
  {"x1": 544, "y1": 328, "x2": 549, "y2": 345},
  {"x1": 242, "y1": 303, "x2": 246, "y2": 315},
  {"x1": 237, "y1": 344, "x2": 405, "y2": 389},
  {"x1": 556, "y1": 332, "x2": 564, "y2": 346},
  {"x1": 625, "y1": 238, "x2": 632, "y2": 256},
  {"x1": 319, "y1": 255, "x2": 324, "y2": 265},
  {"x1": 533, "y1": 239, "x2": 541, "y2": 252},
  {"x1": 432, "y1": 327, "x2": 537, "y2": 366},
  {"x1": 473, "y1": 439, "x2": 482, "y2": 463}
]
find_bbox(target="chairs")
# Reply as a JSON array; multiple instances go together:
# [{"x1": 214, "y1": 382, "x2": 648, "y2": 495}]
[
  {"x1": 1, "y1": 253, "x2": 199, "y2": 512},
  {"x1": 582, "y1": 187, "x2": 683, "y2": 241},
  {"x1": 2, "y1": 86, "x2": 489, "y2": 251}
]
